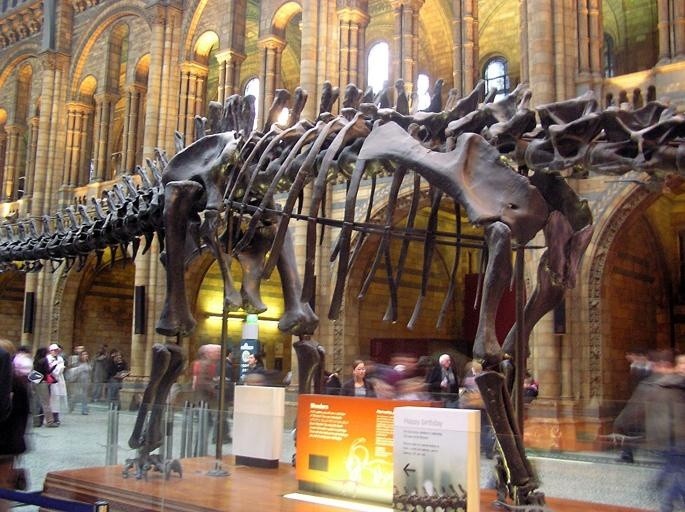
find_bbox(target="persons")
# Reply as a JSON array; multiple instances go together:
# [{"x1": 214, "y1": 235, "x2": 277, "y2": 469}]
[
  {"x1": 191, "y1": 343, "x2": 271, "y2": 445},
  {"x1": 610, "y1": 347, "x2": 685, "y2": 510},
  {"x1": 325, "y1": 351, "x2": 538, "y2": 460},
  {"x1": 0, "y1": 337, "x2": 130, "y2": 511}
]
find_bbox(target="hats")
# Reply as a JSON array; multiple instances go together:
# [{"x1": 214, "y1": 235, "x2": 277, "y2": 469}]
[{"x1": 49, "y1": 344, "x2": 60, "y2": 352}]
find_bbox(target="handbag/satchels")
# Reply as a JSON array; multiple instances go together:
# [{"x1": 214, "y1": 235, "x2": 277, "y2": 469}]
[
  {"x1": 27, "y1": 370, "x2": 44, "y2": 384},
  {"x1": 8, "y1": 455, "x2": 26, "y2": 490},
  {"x1": 46, "y1": 375, "x2": 56, "y2": 384}
]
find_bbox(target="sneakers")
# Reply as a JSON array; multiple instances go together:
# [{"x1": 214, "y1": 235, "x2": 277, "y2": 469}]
[{"x1": 47, "y1": 423, "x2": 57, "y2": 427}]
